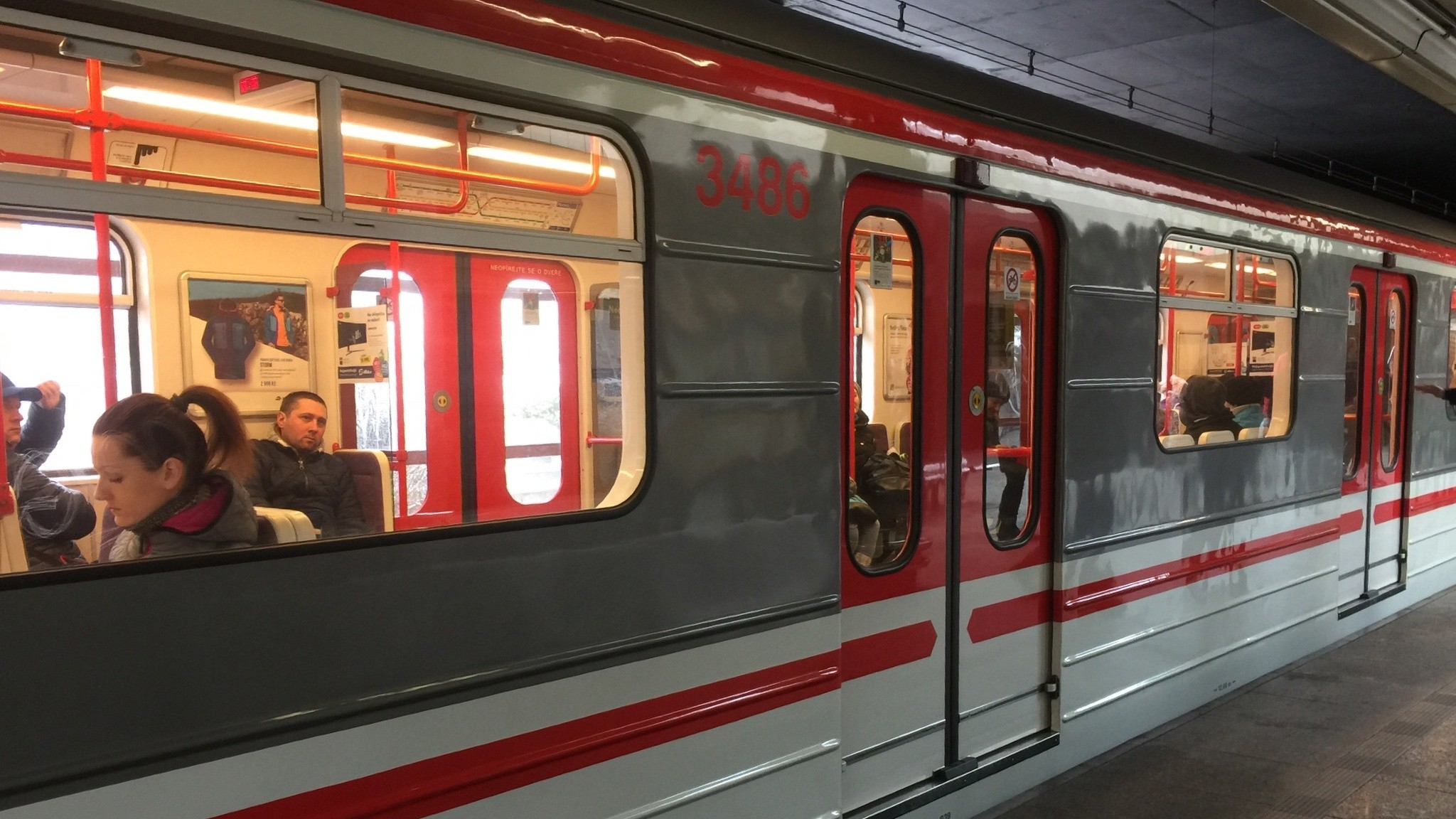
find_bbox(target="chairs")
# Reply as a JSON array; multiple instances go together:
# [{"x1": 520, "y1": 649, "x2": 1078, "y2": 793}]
[
  {"x1": 333, "y1": 449, "x2": 395, "y2": 534},
  {"x1": 254, "y1": 505, "x2": 317, "y2": 544},
  {"x1": 866, "y1": 423, "x2": 889, "y2": 453},
  {"x1": 1159, "y1": 428, "x2": 1269, "y2": 449},
  {"x1": 894, "y1": 421, "x2": 910, "y2": 454},
  {"x1": 0, "y1": 484, "x2": 29, "y2": 575}
]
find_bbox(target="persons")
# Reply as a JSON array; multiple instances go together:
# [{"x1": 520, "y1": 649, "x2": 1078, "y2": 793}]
[
  {"x1": 1416, "y1": 384, "x2": 1456, "y2": 405},
  {"x1": 987, "y1": 371, "x2": 1010, "y2": 447},
  {"x1": 263, "y1": 294, "x2": 295, "y2": 352},
  {"x1": 987, "y1": 342, "x2": 1028, "y2": 541},
  {"x1": 854, "y1": 382, "x2": 878, "y2": 496},
  {"x1": 242, "y1": 391, "x2": 366, "y2": 539},
  {"x1": 0, "y1": 373, "x2": 97, "y2": 571},
  {"x1": 90, "y1": 384, "x2": 258, "y2": 561},
  {"x1": 1178, "y1": 375, "x2": 1268, "y2": 445}
]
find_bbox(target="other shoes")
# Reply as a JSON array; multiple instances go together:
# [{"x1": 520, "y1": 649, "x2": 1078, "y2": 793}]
[{"x1": 996, "y1": 514, "x2": 1021, "y2": 540}]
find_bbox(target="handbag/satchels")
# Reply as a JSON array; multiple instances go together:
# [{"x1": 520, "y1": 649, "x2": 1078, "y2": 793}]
[{"x1": 847, "y1": 495, "x2": 878, "y2": 525}]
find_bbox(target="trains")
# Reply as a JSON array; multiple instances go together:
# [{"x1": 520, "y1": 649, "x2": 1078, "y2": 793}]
[{"x1": 0, "y1": 0, "x2": 1456, "y2": 819}]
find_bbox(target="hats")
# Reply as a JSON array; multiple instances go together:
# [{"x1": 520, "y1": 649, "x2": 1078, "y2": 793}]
[
  {"x1": 1, "y1": 373, "x2": 43, "y2": 402},
  {"x1": 987, "y1": 371, "x2": 1012, "y2": 404},
  {"x1": 1178, "y1": 375, "x2": 1229, "y2": 415},
  {"x1": 1226, "y1": 375, "x2": 1265, "y2": 406},
  {"x1": 854, "y1": 382, "x2": 862, "y2": 400}
]
